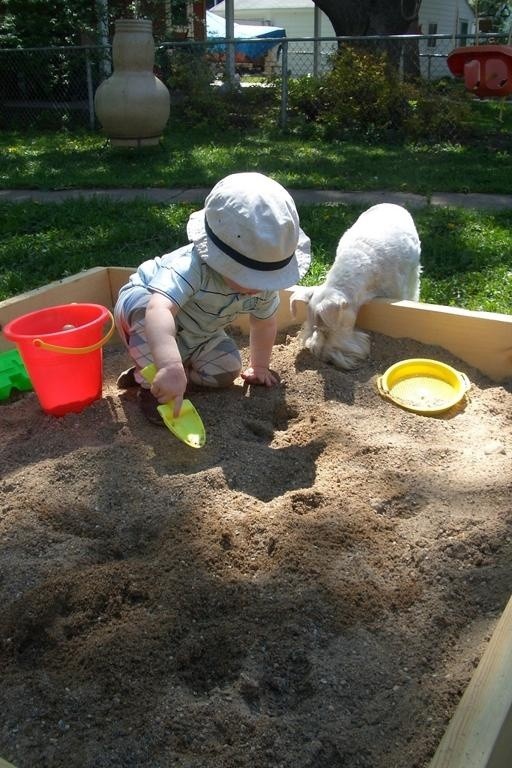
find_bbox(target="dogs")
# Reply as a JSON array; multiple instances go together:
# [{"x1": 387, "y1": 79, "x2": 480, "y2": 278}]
[{"x1": 288, "y1": 202, "x2": 424, "y2": 371}]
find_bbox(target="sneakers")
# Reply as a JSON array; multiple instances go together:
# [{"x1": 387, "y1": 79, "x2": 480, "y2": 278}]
[
  {"x1": 144, "y1": 392, "x2": 163, "y2": 426},
  {"x1": 117, "y1": 366, "x2": 136, "y2": 388}
]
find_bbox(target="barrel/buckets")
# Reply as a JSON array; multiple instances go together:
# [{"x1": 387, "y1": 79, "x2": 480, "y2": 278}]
[{"x1": 3, "y1": 304, "x2": 114, "y2": 417}]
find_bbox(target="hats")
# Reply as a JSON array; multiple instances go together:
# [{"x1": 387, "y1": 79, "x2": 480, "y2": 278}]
[{"x1": 186, "y1": 172, "x2": 312, "y2": 292}]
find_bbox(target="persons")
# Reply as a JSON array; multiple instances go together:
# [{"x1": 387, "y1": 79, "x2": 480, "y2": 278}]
[{"x1": 116, "y1": 171, "x2": 315, "y2": 428}]
